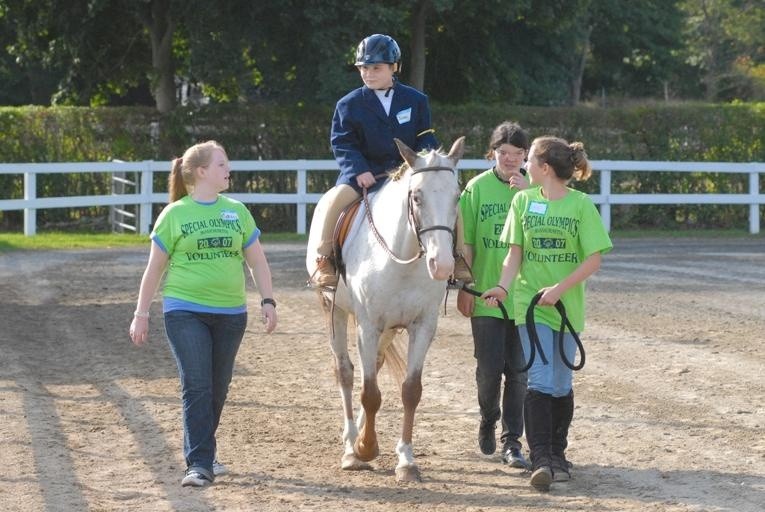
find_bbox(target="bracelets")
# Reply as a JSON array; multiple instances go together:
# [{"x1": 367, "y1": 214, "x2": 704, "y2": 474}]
[
  {"x1": 497, "y1": 284, "x2": 509, "y2": 295},
  {"x1": 260, "y1": 298, "x2": 276, "y2": 308},
  {"x1": 132, "y1": 309, "x2": 151, "y2": 319}
]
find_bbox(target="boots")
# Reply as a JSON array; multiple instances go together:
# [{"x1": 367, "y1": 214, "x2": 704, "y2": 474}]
[
  {"x1": 524, "y1": 389, "x2": 553, "y2": 492},
  {"x1": 553, "y1": 389, "x2": 574, "y2": 481}
]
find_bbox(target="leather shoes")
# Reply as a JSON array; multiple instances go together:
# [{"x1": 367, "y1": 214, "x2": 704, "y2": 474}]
[
  {"x1": 502, "y1": 448, "x2": 525, "y2": 467},
  {"x1": 317, "y1": 258, "x2": 337, "y2": 285},
  {"x1": 455, "y1": 255, "x2": 473, "y2": 282},
  {"x1": 478, "y1": 422, "x2": 497, "y2": 455}
]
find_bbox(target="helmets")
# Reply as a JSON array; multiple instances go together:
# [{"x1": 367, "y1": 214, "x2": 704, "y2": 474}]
[{"x1": 354, "y1": 33, "x2": 401, "y2": 65}]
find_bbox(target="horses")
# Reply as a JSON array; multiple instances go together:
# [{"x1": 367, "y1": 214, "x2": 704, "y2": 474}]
[{"x1": 323, "y1": 134, "x2": 468, "y2": 484}]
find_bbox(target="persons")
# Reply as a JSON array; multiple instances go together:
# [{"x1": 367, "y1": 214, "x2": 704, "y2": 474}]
[
  {"x1": 481, "y1": 135, "x2": 616, "y2": 493},
  {"x1": 126, "y1": 140, "x2": 280, "y2": 488},
  {"x1": 311, "y1": 32, "x2": 473, "y2": 286},
  {"x1": 456, "y1": 121, "x2": 541, "y2": 468}
]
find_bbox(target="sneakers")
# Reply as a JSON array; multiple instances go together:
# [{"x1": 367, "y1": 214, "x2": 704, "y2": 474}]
[
  {"x1": 213, "y1": 462, "x2": 229, "y2": 475},
  {"x1": 182, "y1": 473, "x2": 213, "y2": 488}
]
